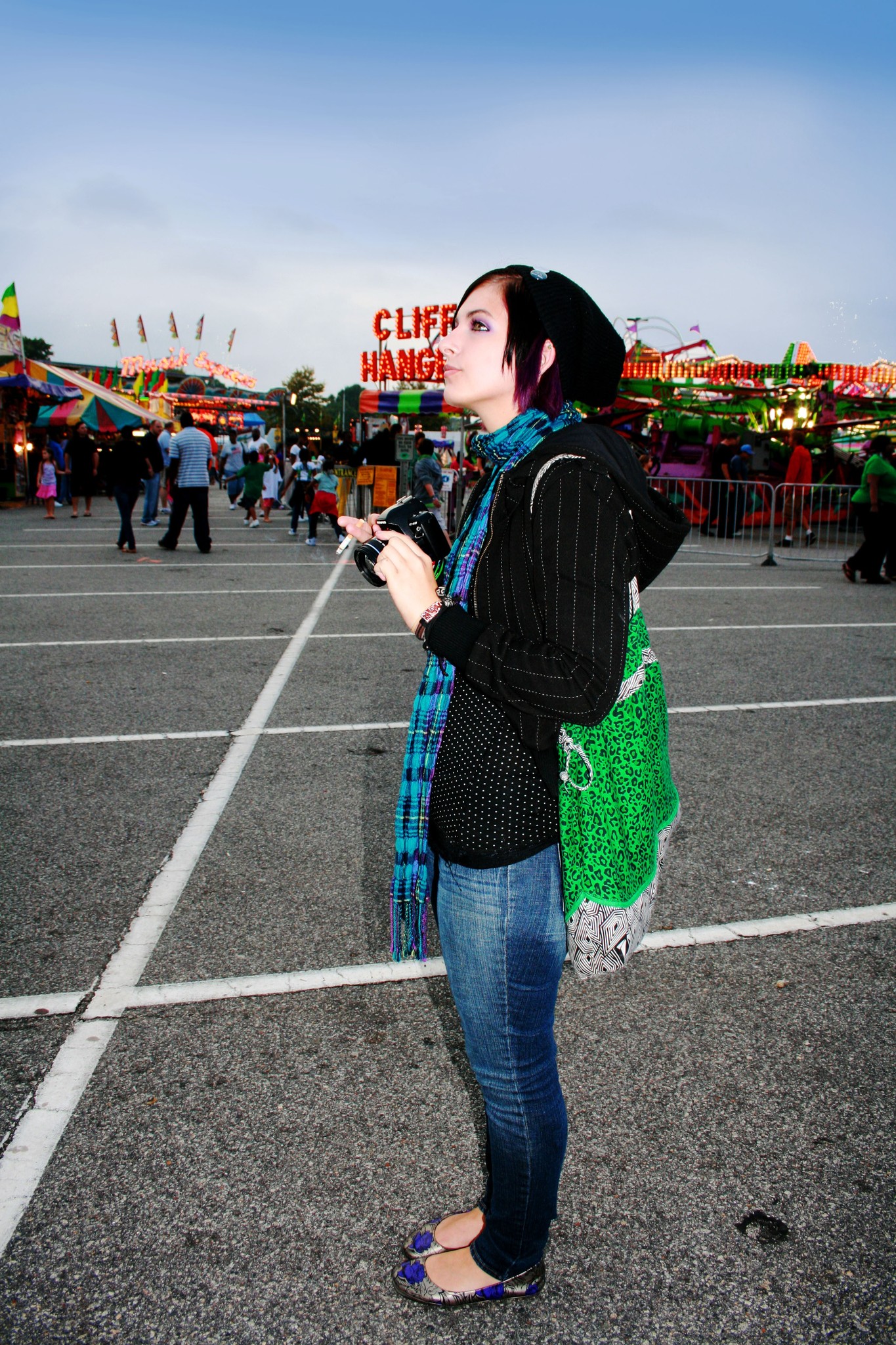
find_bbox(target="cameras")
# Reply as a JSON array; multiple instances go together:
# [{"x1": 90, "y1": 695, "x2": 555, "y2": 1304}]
[{"x1": 350, "y1": 491, "x2": 453, "y2": 590}]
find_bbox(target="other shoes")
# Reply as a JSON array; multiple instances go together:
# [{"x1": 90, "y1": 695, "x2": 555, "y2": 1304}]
[
  {"x1": 699, "y1": 527, "x2": 715, "y2": 537},
  {"x1": 805, "y1": 532, "x2": 816, "y2": 545},
  {"x1": 775, "y1": 539, "x2": 793, "y2": 547},
  {"x1": 391, "y1": 1254, "x2": 546, "y2": 1307},
  {"x1": 734, "y1": 531, "x2": 742, "y2": 536},
  {"x1": 883, "y1": 562, "x2": 896, "y2": 581},
  {"x1": 305, "y1": 537, "x2": 316, "y2": 546},
  {"x1": 44, "y1": 484, "x2": 295, "y2": 553},
  {"x1": 865, "y1": 575, "x2": 890, "y2": 585},
  {"x1": 841, "y1": 563, "x2": 856, "y2": 582},
  {"x1": 298, "y1": 515, "x2": 306, "y2": 522},
  {"x1": 402, "y1": 1210, "x2": 471, "y2": 1259},
  {"x1": 339, "y1": 534, "x2": 349, "y2": 549},
  {"x1": 860, "y1": 572, "x2": 867, "y2": 580},
  {"x1": 717, "y1": 533, "x2": 734, "y2": 539}
]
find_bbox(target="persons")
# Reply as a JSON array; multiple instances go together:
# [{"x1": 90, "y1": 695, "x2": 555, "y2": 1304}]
[
  {"x1": 337, "y1": 263, "x2": 690, "y2": 1306},
  {"x1": 0, "y1": 410, "x2": 485, "y2": 554},
  {"x1": 638, "y1": 454, "x2": 664, "y2": 494},
  {"x1": 704, "y1": 424, "x2": 896, "y2": 584}
]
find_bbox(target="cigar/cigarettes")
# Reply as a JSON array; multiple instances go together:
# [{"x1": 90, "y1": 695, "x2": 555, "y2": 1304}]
[{"x1": 336, "y1": 519, "x2": 365, "y2": 556}]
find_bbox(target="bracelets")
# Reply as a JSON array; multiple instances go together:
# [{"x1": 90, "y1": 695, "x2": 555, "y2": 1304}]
[
  {"x1": 479, "y1": 469, "x2": 484, "y2": 473},
  {"x1": 431, "y1": 495, "x2": 436, "y2": 500},
  {"x1": 654, "y1": 487, "x2": 657, "y2": 490},
  {"x1": 410, "y1": 601, "x2": 444, "y2": 641}
]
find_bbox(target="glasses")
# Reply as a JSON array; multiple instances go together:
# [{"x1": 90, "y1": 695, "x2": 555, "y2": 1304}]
[{"x1": 734, "y1": 439, "x2": 739, "y2": 443}]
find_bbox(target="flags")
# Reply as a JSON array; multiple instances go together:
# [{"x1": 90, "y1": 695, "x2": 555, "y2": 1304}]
[{"x1": 0, "y1": 285, "x2": 23, "y2": 354}]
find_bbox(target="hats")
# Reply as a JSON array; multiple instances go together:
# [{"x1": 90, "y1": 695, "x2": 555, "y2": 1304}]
[{"x1": 741, "y1": 444, "x2": 756, "y2": 454}]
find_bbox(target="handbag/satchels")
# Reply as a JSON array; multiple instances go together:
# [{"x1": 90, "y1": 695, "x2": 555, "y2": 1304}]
[{"x1": 530, "y1": 452, "x2": 681, "y2": 981}]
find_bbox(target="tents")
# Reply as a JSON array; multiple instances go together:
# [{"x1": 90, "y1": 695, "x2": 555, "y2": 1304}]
[
  {"x1": 0, "y1": 356, "x2": 181, "y2": 437},
  {"x1": 356, "y1": 388, "x2": 480, "y2": 540},
  {"x1": 0, "y1": 369, "x2": 84, "y2": 498}
]
